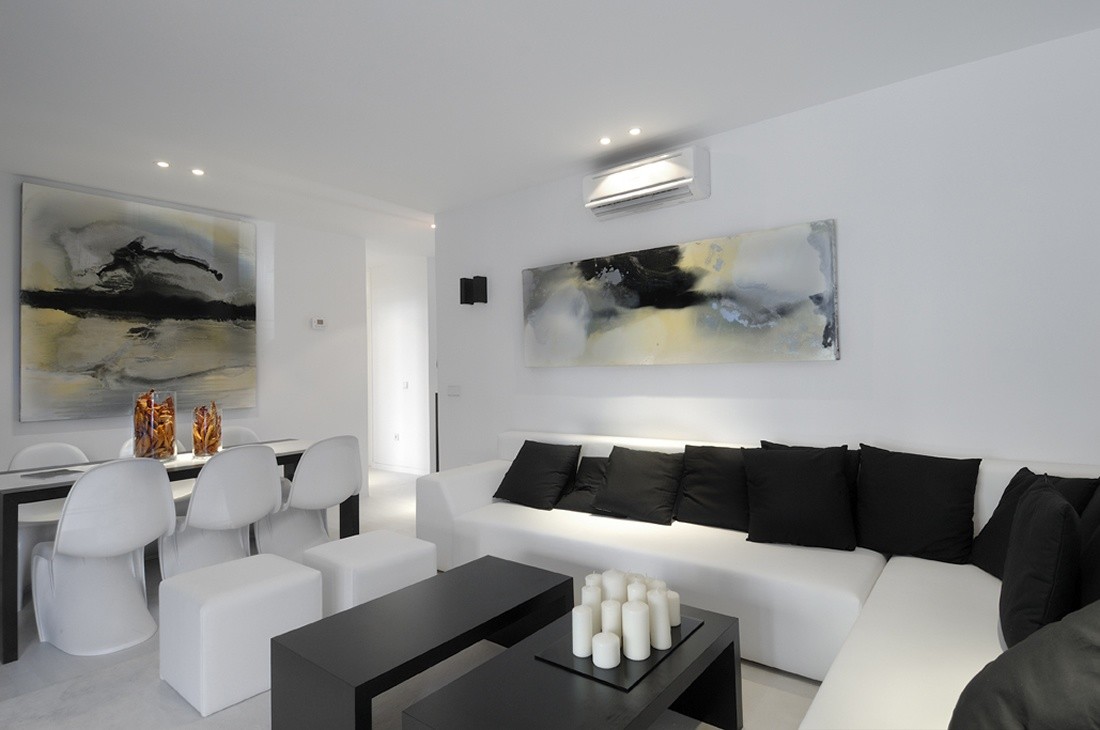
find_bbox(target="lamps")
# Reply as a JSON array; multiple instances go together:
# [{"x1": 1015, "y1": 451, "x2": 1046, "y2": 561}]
[{"x1": 460, "y1": 276, "x2": 487, "y2": 305}]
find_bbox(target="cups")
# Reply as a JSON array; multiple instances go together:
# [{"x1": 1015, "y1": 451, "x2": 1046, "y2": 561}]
[
  {"x1": 131, "y1": 392, "x2": 177, "y2": 462},
  {"x1": 191, "y1": 402, "x2": 223, "y2": 457}
]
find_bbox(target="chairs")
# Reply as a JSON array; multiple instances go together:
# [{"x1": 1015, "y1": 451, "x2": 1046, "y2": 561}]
[{"x1": 7, "y1": 426, "x2": 361, "y2": 656}]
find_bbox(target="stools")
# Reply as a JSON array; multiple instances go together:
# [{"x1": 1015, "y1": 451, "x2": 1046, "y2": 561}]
[
  {"x1": 303, "y1": 528, "x2": 437, "y2": 617},
  {"x1": 159, "y1": 552, "x2": 322, "y2": 715}
]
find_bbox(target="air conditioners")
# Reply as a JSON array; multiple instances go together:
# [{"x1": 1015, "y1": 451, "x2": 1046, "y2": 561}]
[{"x1": 581, "y1": 145, "x2": 711, "y2": 220}]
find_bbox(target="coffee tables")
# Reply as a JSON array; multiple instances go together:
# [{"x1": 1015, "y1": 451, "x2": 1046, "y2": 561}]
[
  {"x1": 401, "y1": 604, "x2": 743, "y2": 730},
  {"x1": 272, "y1": 554, "x2": 574, "y2": 730}
]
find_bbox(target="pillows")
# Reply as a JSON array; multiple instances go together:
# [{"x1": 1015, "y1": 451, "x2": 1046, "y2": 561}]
[
  {"x1": 948, "y1": 466, "x2": 1100, "y2": 730},
  {"x1": 493, "y1": 440, "x2": 982, "y2": 564}
]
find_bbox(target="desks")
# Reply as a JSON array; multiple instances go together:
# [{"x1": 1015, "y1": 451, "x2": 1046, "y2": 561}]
[{"x1": 0, "y1": 438, "x2": 359, "y2": 665}]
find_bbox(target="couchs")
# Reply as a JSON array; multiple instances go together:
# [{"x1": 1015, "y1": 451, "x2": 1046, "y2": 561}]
[
  {"x1": 415, "y1": 431, "x2": 890, "y2": 682},
  {"x1": 798, "y1": 459, "x2": 1100, "y2": 730}
]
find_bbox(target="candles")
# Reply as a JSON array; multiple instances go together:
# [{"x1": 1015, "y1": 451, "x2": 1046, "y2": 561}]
[{"x1": 572, "y1": 568, "x2": 681, "y2": 669}]
